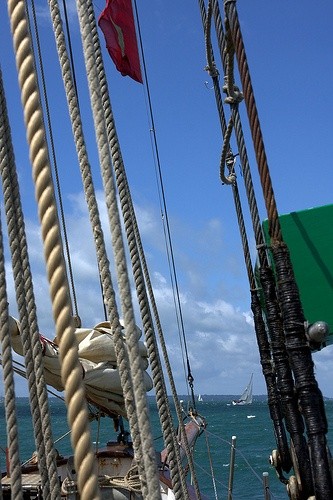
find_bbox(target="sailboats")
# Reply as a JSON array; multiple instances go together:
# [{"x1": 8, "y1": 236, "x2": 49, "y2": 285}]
[{"x1": 227, "y1": 373, "x2": 254, "y2": 406}]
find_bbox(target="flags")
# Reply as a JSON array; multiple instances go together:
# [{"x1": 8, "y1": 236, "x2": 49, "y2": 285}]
[{"x1": 96, "y1": 0, "x2": 143, "y2": 84}]
